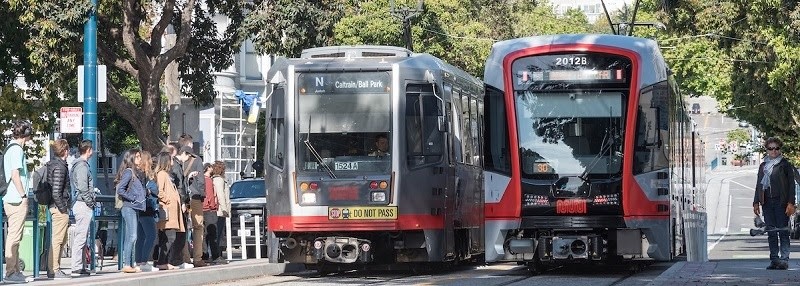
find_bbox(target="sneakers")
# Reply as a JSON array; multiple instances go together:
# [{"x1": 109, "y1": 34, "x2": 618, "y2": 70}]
[
  {"x1": 3, "y1": 272, "x2": 35, "y2": 283},
  {"x1": 48, "y1": 270, "x2": 72, "y2": 279}
]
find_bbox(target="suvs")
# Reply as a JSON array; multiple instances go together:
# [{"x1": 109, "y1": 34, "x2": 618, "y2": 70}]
[{"x1": 217, "y1": 176, "x2": 268, "y2": 258}]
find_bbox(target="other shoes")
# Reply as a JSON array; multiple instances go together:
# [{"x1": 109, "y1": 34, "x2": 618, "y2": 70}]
[
  {"x1": 194, "y1": 260, "x2": 210, "y2": 267},
  {"x1": 122, "y1": 265, "x2": 140, "y2": 273},
  {"x1": 179, "y1": 261, "x2": 194, "y2": 269},
  {"x1": 766, "y1": 260, "x2": 780, "y2": 269},
  {"x1": 778, "y1": 262, "x2": 788, "y2": 269},
  {"x1": 211, "y1": 256, "x2": 230, "y2": 264},
  {"x1": 155, "y1": 263, "x2": 174, "y2": 270},
  {"x1": 71, "y1": 269, "x2": 96, "y2": 276},
  {"x1": 140, "y1": 264, "x2": 159, "y2": 272}
]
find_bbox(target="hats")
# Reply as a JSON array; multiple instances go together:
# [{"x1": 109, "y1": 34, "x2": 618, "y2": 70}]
[
  {"x1": 203, "y1": 163, "x2": 214, "y2": 172},
  {"x1": 180, "y1": 146, "x2": 197, "y2": 158}
]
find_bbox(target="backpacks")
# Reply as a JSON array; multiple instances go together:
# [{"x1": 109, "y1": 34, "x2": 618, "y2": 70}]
[
  {"x1": 32, "y1": 159, "x2": 61, "y2": 206},
  {"x1": 65, "y1": 159, "x2": 89, "y2": 209},
  {"x1": 115, "y1": 167, "x2": 135, "y2": 209},
  {"x1": 0, "y1": 142, "x2": 25, "y2": 196}
]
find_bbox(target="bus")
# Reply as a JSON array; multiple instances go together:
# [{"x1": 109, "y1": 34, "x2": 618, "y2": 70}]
[
  {"x1": 483, "y1": 0, "x2": 709, "y2": 276},
  {"x1": 246, "y1": 0, "x2": 483, "y2": 276}
]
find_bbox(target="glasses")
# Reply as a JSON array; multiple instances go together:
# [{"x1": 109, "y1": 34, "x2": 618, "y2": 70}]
[
  {"x1": 136, "y1": 154, "x2": 141, "y2": 159},
  {"x1": 767, "y1": 147, "x2": 781, "y2": 151}
]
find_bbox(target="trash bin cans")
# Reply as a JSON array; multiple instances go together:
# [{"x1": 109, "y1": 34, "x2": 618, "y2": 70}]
[{"x1": 18, "y1": 221, "x2": 45, "y2": 272}]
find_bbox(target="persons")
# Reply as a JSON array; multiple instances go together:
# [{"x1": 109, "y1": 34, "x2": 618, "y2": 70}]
[
  {"x1": 2, "y1": 121, "x2": 230, "y2": 283},
  {"x1": 753, "y1": 138, "x2": 796, "y2": 270},
  {"x1": 367, "y1": 135, "x2": 392, "y2": 174}
]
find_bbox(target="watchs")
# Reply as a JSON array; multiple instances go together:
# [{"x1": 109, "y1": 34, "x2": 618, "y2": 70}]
[{"x1": 22, "y1": 194, "x2": 26, "y2": 199}]
